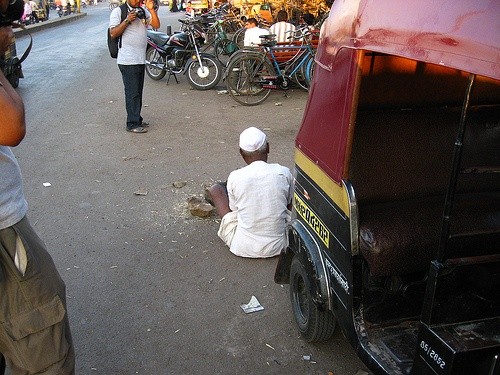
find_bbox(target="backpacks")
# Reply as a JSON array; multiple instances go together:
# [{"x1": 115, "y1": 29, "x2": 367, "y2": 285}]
[{"x1": 108, "y1": 4, "x2": 128, "y2": 57}]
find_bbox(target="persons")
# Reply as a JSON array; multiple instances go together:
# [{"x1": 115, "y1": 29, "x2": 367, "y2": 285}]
[
  {"x1": 110, "y1": 0, "x2": 160, "y2": 132},
  {"x1": 270, "y1": 9, "x2": 296, "y2": 82},
  {"x1": 244, "y1": 18, "x2": 270, "y2": 77},
  {"x1": 300, "y1": 14, "x2": 315, "y2": 42},
  {"x1": 218, "y1": 0, "x2": 229, "y2": 12},
  {"x1": 21, "y1": 0, "x2": 49, "y2": 23},
  {"x1": 186, "y1": 2, "x2": 193, "y2": 12},
  {"x1": 210, "y1": 127, "x2": 294, "y2": 258},
  {"x1": 55, "y1": 0, "x2": 76, "y2": 15},
  {"x1": 0, "y1": 0, "x2": 76, "y2": 375}
]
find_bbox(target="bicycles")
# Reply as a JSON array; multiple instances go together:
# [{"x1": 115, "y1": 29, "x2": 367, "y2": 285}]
[{"x1": 179, "y1": 4, "x2": 315, "y2": 107}]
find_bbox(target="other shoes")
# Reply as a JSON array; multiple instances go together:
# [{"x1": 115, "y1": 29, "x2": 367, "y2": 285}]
[
  {"x1": 126, "y1": 126, "x2": 147, "y2": 132},
  {"x1": 139, "y1": 120, "x2": 149, "y2": 127}
]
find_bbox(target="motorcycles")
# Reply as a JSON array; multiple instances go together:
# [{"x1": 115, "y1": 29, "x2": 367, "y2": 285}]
[
  {"x1": 273, "y1": 0, "x2": 500, "y2": 375},
  {"x1": 145, "y1": 18, "x2": 222, "y2": 90}
]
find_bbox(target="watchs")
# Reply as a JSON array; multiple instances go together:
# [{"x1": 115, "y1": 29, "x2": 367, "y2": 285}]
[{"x1": 125, "y1": 19, "x2": 130, "y2": 24}]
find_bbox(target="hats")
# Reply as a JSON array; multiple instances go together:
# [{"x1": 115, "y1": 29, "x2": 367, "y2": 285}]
[{"x1": 239, "y1": 127, "x2": 266, "y2": 151}]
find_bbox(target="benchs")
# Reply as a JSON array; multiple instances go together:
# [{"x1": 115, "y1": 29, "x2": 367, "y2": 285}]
[
  {"x1": 256, "y1": 39, "x2": 319, "y2": 69},
  {"x1": 354, "y1": 104, "x2": 500, "y2": 285}
]
